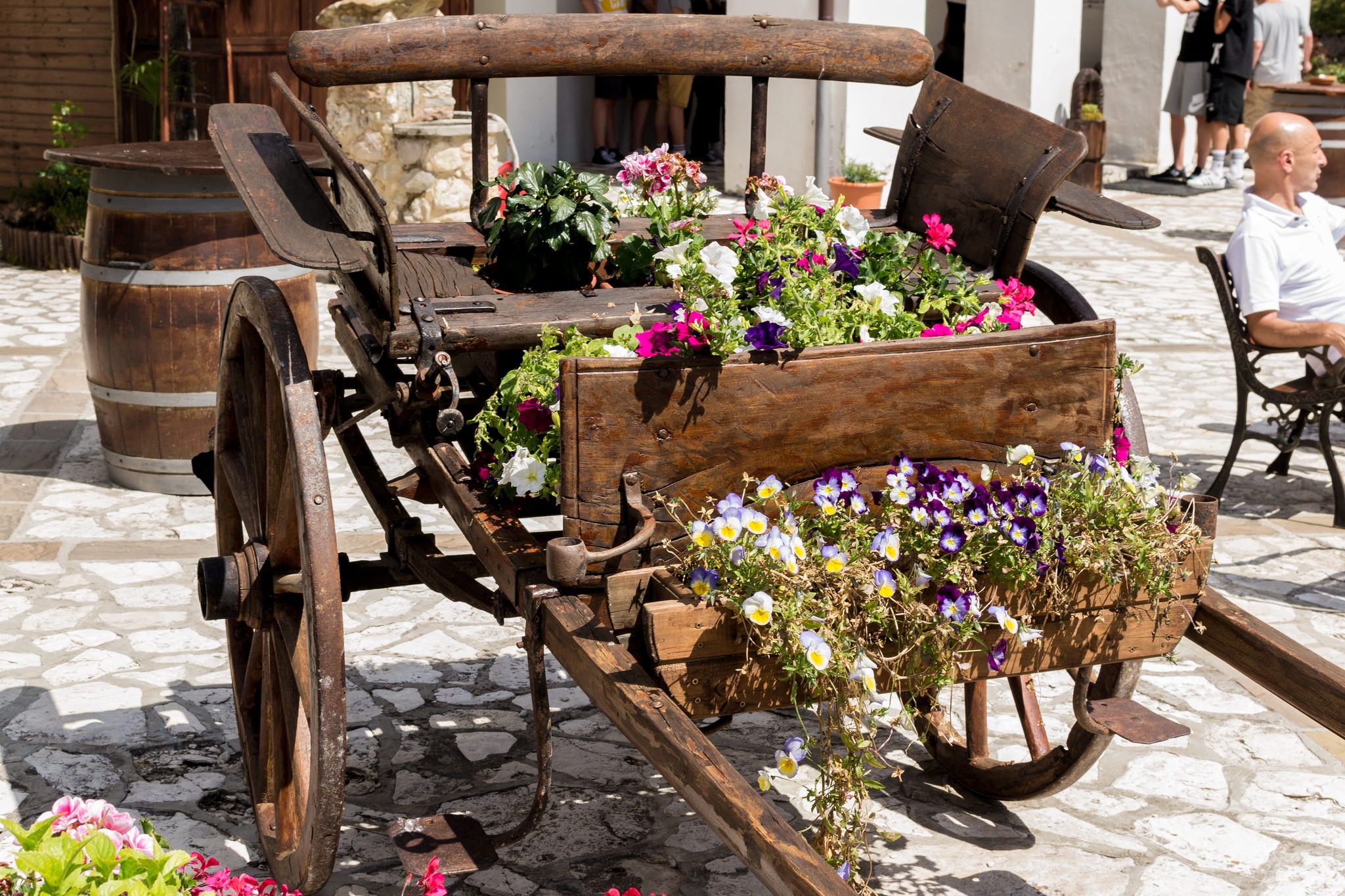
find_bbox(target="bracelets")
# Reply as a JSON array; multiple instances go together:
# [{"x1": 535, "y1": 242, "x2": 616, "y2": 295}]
[{"x1": 1218, "y1": 0, "x2": 1224, "y2": 4}]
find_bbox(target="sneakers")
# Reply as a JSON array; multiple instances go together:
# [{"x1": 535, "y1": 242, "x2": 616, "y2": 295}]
[
  {"x1": 590, "y1": 146, "x2": 623, "y2": 167},
  {"x1": 1151, "y1": 164, "x2": 1187, "y2": 185},
  {"x1": 1221, "y1": 171, "x2": 1244, "y2": 188},
  {"x1": 1186, "y1": 166, "x2": 1201, "y2": 180},
  {"x1": 1187, "y1": 169, "x2": 1226, "y2": 189},
  {"x1": 608, "y1": 148, "x2": 625, "y2": 162}
]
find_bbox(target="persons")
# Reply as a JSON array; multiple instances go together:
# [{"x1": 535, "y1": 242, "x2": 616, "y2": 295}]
[
  {"x1": 1245, "y1": 0, "x2": 1313, "y2": 169},
  {"x1": 1188, "y1": 0, "x2": 1254, "y2": 189},
  {"x1": 581, "y1": 0, "x2": 726, "y2": 169},
  {"x1": 1227, "y1": 111, "x2": 1345, "y2": 389},
  {"x1": 1149, "y1": 0, "x2": 1219, "y2": 183}
]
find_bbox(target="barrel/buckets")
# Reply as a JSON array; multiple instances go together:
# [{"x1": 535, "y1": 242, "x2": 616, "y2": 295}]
[{"x1": 80, "y1": 167, "x2": 320, "y2": 494}]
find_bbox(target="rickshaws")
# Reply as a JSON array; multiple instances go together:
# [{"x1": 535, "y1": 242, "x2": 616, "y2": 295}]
[{"x1": 191, "y1": 13, "x2": 1345, "y2": 896}]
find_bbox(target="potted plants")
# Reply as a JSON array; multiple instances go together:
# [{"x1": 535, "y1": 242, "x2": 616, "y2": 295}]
[{"x1": 827, "y1": 147, "x2": 888, "y2": 210}]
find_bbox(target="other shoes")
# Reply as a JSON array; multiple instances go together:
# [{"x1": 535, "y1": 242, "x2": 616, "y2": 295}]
[{"x1": 682, "y1": 152, "x2": 689, "y2": 161}]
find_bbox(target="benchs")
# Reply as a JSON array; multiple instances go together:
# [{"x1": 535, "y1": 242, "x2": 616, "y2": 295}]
[{"x1": 1195, "y1": 245, "x2": 1345, "y2": 530}]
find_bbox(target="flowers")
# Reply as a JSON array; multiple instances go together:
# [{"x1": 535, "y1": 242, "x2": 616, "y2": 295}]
[
  {"x1": 465, "y1": 142, "x2": 1146, "y2": 500},
  {"x1": 664, "y1": 441, "x2": 1208, "y2": 886}
]
